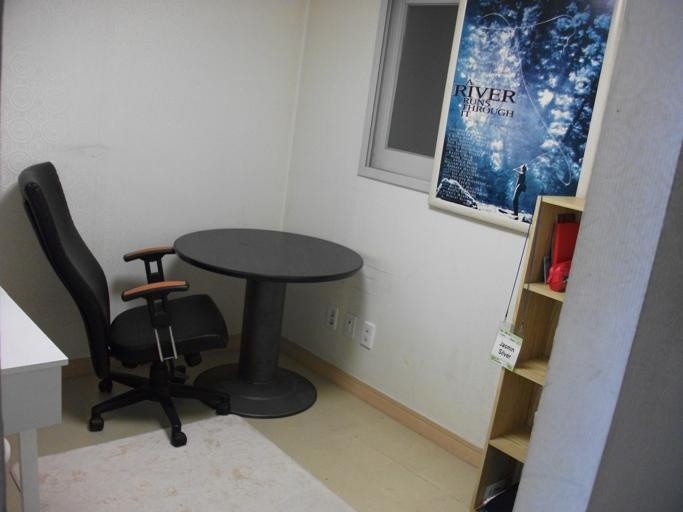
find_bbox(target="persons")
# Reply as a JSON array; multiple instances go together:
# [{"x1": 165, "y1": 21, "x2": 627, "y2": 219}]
[{"x1": 510, "y1": 163, "x2": 527, "y2": 217}]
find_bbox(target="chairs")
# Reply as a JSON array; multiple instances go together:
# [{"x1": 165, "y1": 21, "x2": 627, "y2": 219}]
[{"x1": 17, "y1": 161, "x2": 231, "y2": 448}]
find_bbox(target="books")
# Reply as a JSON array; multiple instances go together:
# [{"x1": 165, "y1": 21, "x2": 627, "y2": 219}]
[
  {"x1": 555, "y1": 211, "x2": 576, "y2": 224},
  {"x1": 552, "y1": 220, "x2": 580, "y2": 264}
]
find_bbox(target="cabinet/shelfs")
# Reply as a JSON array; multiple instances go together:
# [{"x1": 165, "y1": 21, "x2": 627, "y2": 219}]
[{"x1": 469, "y1": 194, "x2": 584, "y2": 512}]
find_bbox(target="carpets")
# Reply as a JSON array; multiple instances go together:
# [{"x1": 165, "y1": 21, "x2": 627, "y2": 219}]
[{"x1": 9, "y1": 414, "x2": 355, "y2": 512}]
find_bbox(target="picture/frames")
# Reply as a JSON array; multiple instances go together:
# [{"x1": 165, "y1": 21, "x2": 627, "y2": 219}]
[{"x1": 427, "y1": 0, "x2": 623, "y2": 235}]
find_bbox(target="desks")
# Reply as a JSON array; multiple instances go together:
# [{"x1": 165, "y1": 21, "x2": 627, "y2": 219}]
[
  {"x1": 0, "y1": 286, "x2": 67, "y2": 512},
  {"x1": 171, "y1": 228, "x2": 363, "y2": 420}
]
